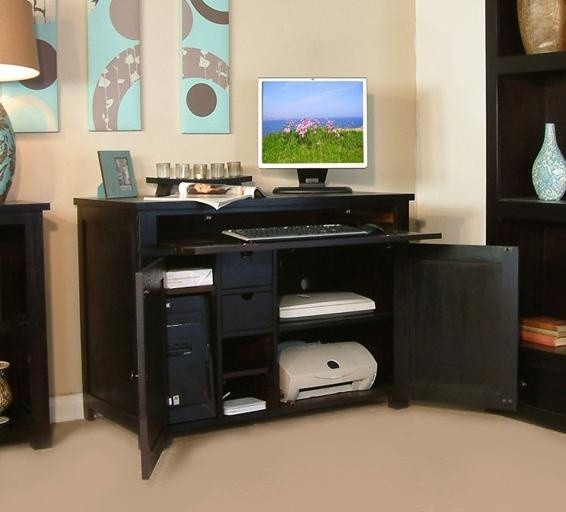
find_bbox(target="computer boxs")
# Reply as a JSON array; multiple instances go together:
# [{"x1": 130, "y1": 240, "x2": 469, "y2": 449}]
[{"x1": 163, "y1": 294, "x2": 218, "y2": 424}]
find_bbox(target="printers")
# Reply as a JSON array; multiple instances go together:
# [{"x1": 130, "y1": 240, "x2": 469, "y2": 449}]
[{"x1": 279, "y1": 341, "x2": 378, "y2": 403}]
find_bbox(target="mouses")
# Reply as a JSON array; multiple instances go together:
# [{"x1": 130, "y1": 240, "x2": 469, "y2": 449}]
[{"x1": 363, "y1": 222, "x2": 387, "y2": 234}]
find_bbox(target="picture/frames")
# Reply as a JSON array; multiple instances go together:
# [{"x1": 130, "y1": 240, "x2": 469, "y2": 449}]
[{"x1": 98, "y1": 149, "x2": 139, "y2": 199}]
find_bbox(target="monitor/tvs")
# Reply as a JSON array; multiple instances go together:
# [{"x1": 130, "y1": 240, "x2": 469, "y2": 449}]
[{"x1": 256, "y1": 77, "x2": 368, "y2": 194}]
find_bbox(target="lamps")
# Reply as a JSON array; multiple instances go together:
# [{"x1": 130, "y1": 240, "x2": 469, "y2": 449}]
[{"x1": 0, "y1": 1, "x2": 41, "y2": 205}]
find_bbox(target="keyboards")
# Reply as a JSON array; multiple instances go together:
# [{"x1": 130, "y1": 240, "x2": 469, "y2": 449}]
[{"x1": 221, "y1": 223, "x2": 368, "y2": 243}]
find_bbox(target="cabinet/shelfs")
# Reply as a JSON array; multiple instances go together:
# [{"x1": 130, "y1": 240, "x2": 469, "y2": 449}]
[
  {"x1": 69, "y1": 191, "x2": 521, "y2": 481},
  {"x1": 1, "y1": 198, "x2": 53, "y2": 450},
  {"x1": 483, "y1": 1, "x2": 566, "y2": 435}
]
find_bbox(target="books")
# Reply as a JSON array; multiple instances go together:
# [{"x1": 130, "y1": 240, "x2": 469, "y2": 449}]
[{"x1": 143, "y1": 181, "x2": 273, "y2": 211}]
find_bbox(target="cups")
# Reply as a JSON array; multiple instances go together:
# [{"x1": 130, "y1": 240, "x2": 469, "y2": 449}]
[
  {"x1": 210, "y1": 162, "x2": 225, "y2": 179},
  {"x1": 155, "y1": 163, "x2": 170, "y2": 179},
  {"x1": 227, "y1": 161, "x2": 242, "y2": 178},
  {"x1": 192, "y1": 163, "x2": 207, "y2": 180},
  {"x1": 175, "y1": 163, "x2": 190, "y2": 179}
]
